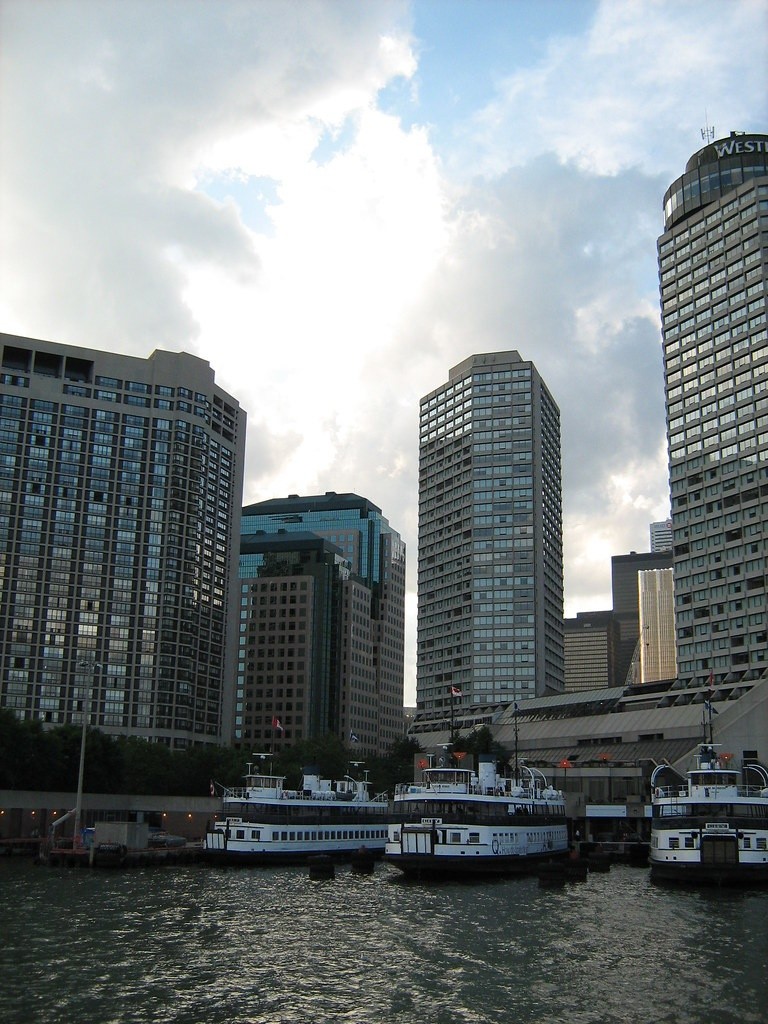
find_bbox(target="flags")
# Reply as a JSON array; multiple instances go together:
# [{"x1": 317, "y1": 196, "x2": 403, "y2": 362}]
[
  {"x1": 514, "y1": 703, "x2": 520, "y2": 712},
  {"x1": 451, "y1": 687, "x2": 462, "y2": 696},
  {"x1": 273, "y1": 719, "x2": 283, "y2": 730},
  {"x1": 210, "y1": 781, "x2": 214, "y2": 796},
  {"x1": 350, "y1": 731, "x2": 358, "y2": 740}
]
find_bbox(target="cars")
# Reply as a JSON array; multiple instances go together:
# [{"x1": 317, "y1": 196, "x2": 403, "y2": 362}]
[{"x1": 147, "y1": 826, "x2": 188, "y2": 848}]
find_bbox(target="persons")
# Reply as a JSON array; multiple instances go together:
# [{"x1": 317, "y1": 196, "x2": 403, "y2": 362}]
[{"x1": 547, "y1": 784, "x2": 553, "y2": 791}]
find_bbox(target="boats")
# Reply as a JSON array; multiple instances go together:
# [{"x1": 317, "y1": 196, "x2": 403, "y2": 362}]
[
  {"x1": 196, "y1": 714, "x2": 393, "y2": 868},
  {"x1": 383, "y1": 685, "x2": 574, "y2": 881},
  {"x1": 643, "y1": 689, "x2": 768, "y2": 886}
]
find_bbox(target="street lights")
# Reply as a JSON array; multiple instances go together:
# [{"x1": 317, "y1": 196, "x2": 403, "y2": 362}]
[{"x1": 69, "y1": 661, "x2": 108, "y2": 849}]
